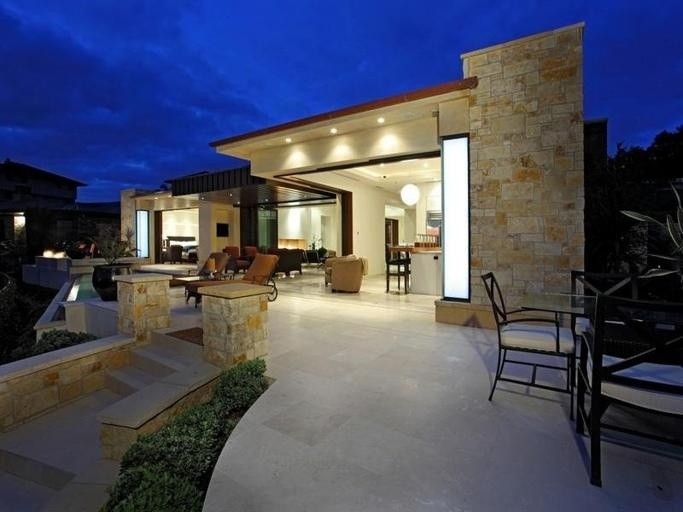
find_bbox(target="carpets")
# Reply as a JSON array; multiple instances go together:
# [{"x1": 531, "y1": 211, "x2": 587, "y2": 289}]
[{"x1": 166, "y1": 327, "x2": 203, "y2": 345}]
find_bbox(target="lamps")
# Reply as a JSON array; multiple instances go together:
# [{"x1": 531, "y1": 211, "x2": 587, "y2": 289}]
[{"x1": 400, "y1": 184, "x2": 420, "y2": 206}]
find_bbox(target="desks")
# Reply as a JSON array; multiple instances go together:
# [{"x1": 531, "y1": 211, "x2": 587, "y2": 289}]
[{"x1": 391, "y1": 247, "x2": 415, "y2": 294}]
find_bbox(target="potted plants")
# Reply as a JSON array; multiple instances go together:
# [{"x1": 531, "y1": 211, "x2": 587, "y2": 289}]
[{"x1": 92, "y1": 223, "x2": 142, "y2": 301}]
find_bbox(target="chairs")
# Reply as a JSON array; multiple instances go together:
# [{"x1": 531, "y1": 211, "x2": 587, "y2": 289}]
[
  {"x1": 577, "y1": 294, "x2": 682, "y2": 486},
  {"x1": 566, "y1": 272, "x2": 635, "y2": 357},
  {"x1": 385, "y1": 245, "x2": 411, "y2": 295},
  {"x1": 169, "y1": 245, "x2": 336, "y2": 309},
  {"x1": 325, "y1": 255, "x2": 364, "y2": 293},
  {"x1": 481, "y1": 272, "x2": 584, "y2": 419}
]
